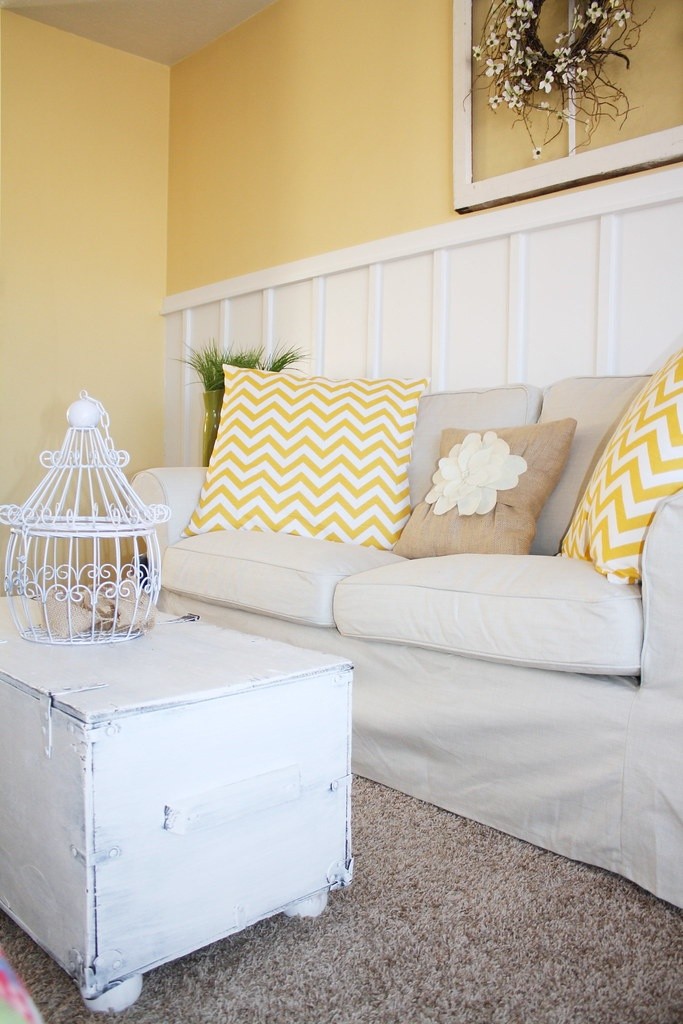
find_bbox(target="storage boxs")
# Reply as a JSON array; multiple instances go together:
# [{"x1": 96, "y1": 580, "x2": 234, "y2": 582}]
[{"x1": 0, "y1": 592, "x2": 353, "y2": 1012}]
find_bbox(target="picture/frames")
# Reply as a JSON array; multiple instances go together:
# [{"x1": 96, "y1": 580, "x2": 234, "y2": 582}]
[{"x1": 452, "y1": 0, "x2": 683, "y2": 216}]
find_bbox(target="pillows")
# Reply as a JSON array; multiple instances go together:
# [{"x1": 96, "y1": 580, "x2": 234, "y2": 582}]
[
  {"x1": 405, "y1": 385, "x2": 547, "y2": 519},
  {"x1": 394, "y1": 417, "x2": 577, "y2": 560},
  {"x1": 532, "y1": 372, "x2": 653, "y2": 560},
  {"x1": 555, "y1": 346, "x2": 683, "y2": 585},
  {"x1": 181, "y1": 363, "x2": 427, "y2": 553}
]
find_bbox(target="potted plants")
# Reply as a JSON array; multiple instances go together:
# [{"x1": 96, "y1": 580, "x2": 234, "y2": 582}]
[{"x1": 169, "y1": 338, "x2": 310, "y2": 468}]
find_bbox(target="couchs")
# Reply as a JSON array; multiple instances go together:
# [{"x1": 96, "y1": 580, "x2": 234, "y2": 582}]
[{"x1": 125, "y1": 371, "x2": 683, "y2": 915}]
